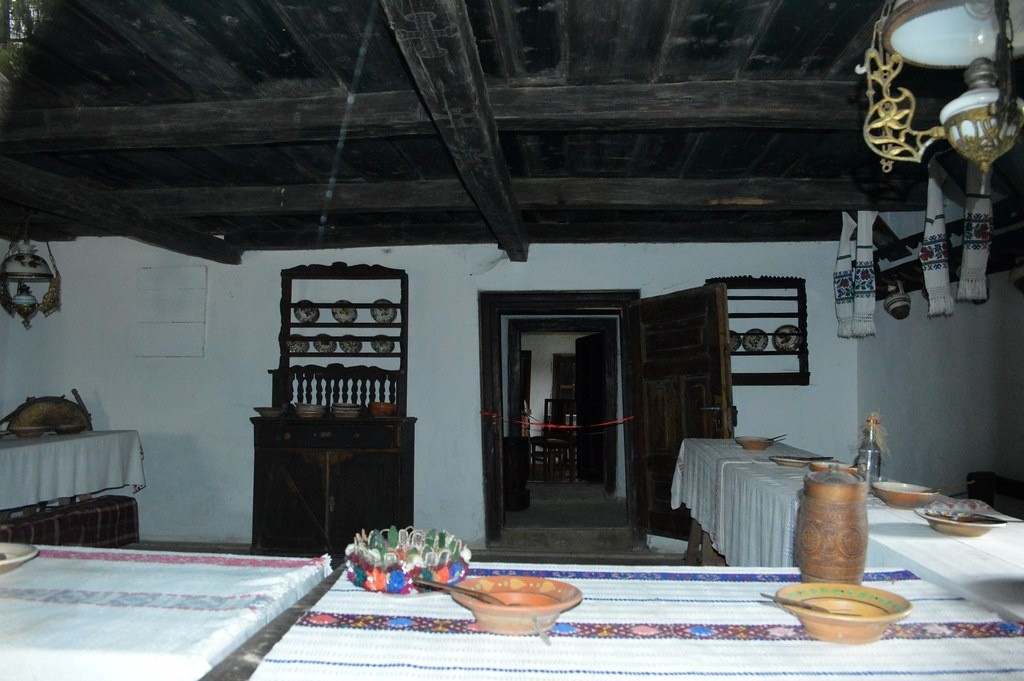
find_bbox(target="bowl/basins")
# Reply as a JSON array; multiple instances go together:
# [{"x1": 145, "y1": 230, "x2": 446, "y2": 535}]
[
  {"x1": 872, "y1": 481, "x2": 939, "y2": 509},
  {"x1": 369, "y1": 402, "x2": 396, "y2": 418},
  {"x1": 332, "y1": 403, "x2": 363, "y2": 418}
]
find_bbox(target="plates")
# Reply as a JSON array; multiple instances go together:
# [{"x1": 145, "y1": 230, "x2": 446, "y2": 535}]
[
  {"x1": 339, "y1": 335, "x2": 362, "y2": 353},
  {"x1": 332, "y1": 300, "x2": 357, "y2": 323},
  {"x1": 769, "y1": 456, "x2": 812, "y2": 467},
  {"x1": 811, "y1": 462, "x2": 858, "y2": 474},
  {"x1": 913, "y1": 508, "x2": 1006, "y2": 537},
  {"x1": 0, "y1": 542, "x2": 38, "y2": 573},
  {"x1": 371, "y1": 299, "x2": 397, "y2": 322},
  {"x1": 294, "y1": 402, "x2": 328, "y2": 419},
  {"x1": 450, "y1": 575, "x2": 583, "y2": 635},
  {"x1": 52, "y1": 425, "x2": 88, "y2": 435},
  {"x1": 294, "y1": 300, "x2": 320, "y2": 323},
  {"x1": 7, "y1": 426, "x2": 50, "y2": 438},
  {"x1": 742, "y1": 328, "x2": 769, "y2": 353},
  {"x1": 371, "y1": 334, "x2": 395, "y2": 353},
  {"x1": 774, "y1": 583, "x2": 914, "y2": 643},
  {"x1": 286, "y1": 334, "x2": 309, "y2": 353},
  {"x1": 253, "y1": 407, "x2": 287, "y2": 417},
  {"x1": 734, "y1": 436, "x2": 774, "y2": 451},
  {"x1": 314, "y1": 334, "x2": 337, "y2": 352},
  {"x1": 772, "y1": 324, "x2": 800, "y2": 351},
  {"x1": 729, "y1": 330, "x2": 742, "y2": 351}
]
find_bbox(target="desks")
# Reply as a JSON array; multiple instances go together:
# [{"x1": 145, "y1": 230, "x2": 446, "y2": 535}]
[{"x1": 0, "y1": 432, "x2": 1024, "y2": 681}]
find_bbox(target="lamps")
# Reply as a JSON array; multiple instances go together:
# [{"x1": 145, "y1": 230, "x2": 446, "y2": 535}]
[{"x1": 852, "y1": 0, "x2": 1024, "y2": 174}]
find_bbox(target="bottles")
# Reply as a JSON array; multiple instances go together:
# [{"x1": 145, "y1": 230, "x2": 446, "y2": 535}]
[{"x1": 854, "y1": 419, "x2": 881, "y2": 495}]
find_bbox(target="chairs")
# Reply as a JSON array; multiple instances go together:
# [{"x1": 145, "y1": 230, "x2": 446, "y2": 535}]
[
  {"x1": 531, "y1": 398, "x2": 576, "y2": 482},
  {"x1": 882, "y1": 277, "x2": 913, "y2": 319}
]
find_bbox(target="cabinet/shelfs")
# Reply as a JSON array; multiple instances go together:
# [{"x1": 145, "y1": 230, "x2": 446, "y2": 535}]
[
  {"x1": 0, "y1": 244, "x2": 63, "y2": 331},
  {"x1": 267, "y1": 261, "x2": 409, "y2": 414},
  {"x1": 706, "y1": 275, "x2": 811, "y2": 386},
  {"x1": 247, "y1": 416, "x2": 419, "y2": 559}
]
